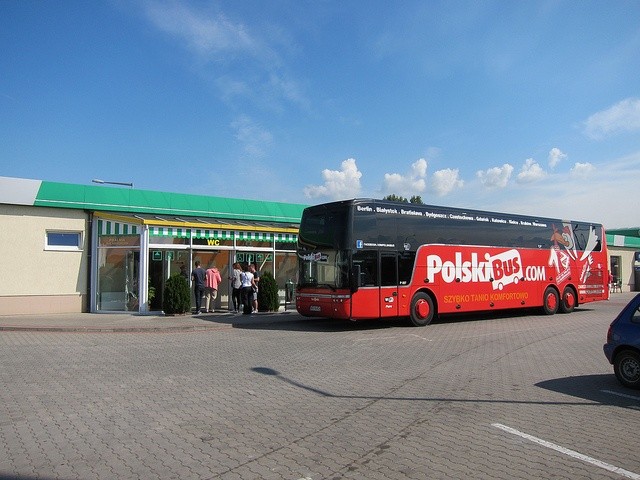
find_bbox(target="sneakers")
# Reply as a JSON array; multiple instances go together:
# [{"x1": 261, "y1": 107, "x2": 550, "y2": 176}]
[{"x1": 251, "y1": 310, "x2": 258, "y2": 314}]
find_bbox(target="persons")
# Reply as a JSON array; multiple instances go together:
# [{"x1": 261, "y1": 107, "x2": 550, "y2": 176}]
[
  {"x1": 228, "y1": 263, "x2": 243, "y2": 312},
  {"x1": 249, "y1": 264, "x2": 260, "y2": 313},
  {"x1": 191, "y1": 260, "x2": 206, "y2": 314},
  {"x1": 204, "y1": 266, "x2": 222, "y2": 312},
  {"x1": 240, "y1": 265, "x2": 258, "y2": 315}
]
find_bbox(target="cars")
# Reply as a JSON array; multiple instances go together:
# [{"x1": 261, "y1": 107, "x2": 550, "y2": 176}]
[{"x1": 603, "y1": 292, "x2": 639, "y2": 390}]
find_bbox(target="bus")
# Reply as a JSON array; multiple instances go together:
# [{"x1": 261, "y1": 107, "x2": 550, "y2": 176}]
[{"x1": 294, "y1": 198, "x2": 609, "y2": 327}]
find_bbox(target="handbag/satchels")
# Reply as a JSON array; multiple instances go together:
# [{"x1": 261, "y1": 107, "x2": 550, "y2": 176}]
[{"x1": 243, "y1": 271, "x2": 256, "y2": 293}]
